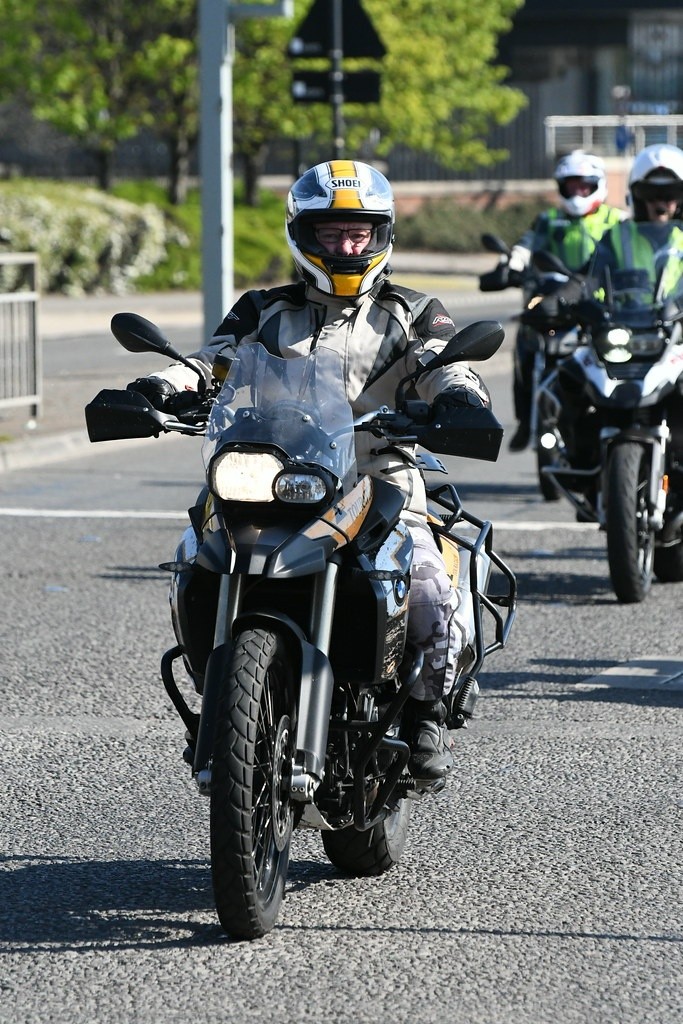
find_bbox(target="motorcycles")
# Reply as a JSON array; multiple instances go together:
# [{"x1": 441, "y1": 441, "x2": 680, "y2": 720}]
[
  {"x1": 82, "y1": 312, "x2": 520, "y2": 943},
  {"x1": 476, "y1": 230, "x2": 603, "y2": 502},
  {"x1": 520, "y1": 248, "x2": 683, "y2": 601}
]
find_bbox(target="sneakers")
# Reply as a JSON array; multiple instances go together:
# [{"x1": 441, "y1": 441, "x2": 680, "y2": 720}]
[
  {"x1": 183, "y1": 747, "x2": 195, "y2": 765},
  {"x1": 402, "y1": 696, "x2": 454, "y2": 779}
]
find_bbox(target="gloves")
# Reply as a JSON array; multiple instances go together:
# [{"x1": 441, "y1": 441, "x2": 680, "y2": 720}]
[{"x1": 126, "y1": 376, "x2": 178, "y2": 438}]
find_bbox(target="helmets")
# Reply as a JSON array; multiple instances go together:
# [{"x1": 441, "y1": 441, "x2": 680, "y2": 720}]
[
  {"x1": 553, "y1": 149, "x2": 608, "y2": 217},
  {"x1": 624, "y1": 144, "x2": 683, "y2": 219},
  {"x1": 285, "y1": 159, "x2": 395, "y2": 298}
]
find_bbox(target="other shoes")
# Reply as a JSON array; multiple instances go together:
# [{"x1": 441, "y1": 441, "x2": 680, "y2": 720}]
[
  {"x1": 509, "y1": 422, "x2": 531, "y2": 451},
  {"x1": 575, "y1": 506, "x2": 599, "y2": 522}
]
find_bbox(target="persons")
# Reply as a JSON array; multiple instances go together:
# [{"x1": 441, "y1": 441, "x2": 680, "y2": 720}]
[
  {"x1": 496, "y1": 150, "x2": 628, "y2": 453},
  {"x1": 126, "y1": 160, "x2": 493, "y2": 777},
  {"x1": 527, "y1": 144, "x2": 683, "y2": 548}
]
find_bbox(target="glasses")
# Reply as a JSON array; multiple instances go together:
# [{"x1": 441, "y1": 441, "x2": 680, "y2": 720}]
[
  {"x1": 314, "y1": 225, "x2": 372, "y2": 244},
  {"x1": 632, "y1": 183, "x2": 683, "y2": 202}
]
